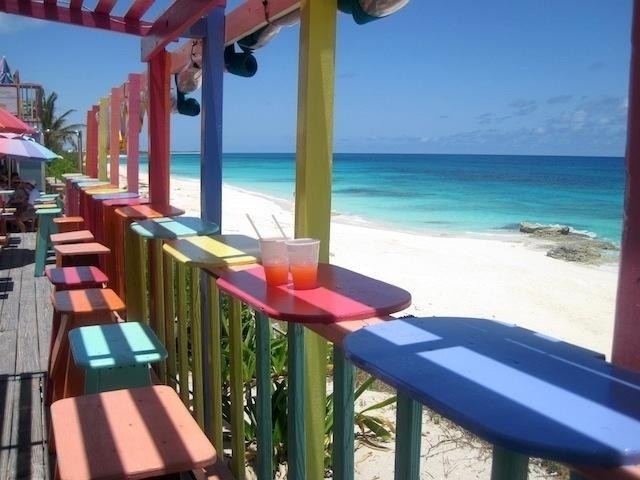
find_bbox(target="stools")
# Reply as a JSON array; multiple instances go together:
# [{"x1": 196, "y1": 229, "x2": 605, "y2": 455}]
[
  {"x1": 45, "y1": 269, "x2": 107, "y2": 362},
  {"x1": 35, "y1": 177, "x2": 112, "y2": 277},
  {"x1": 67, "y1": 320, "x2": 170, "y2": 393},
  {"x1": 49, "y1": 386, "x2": 216, "y2": 480},
  {"x1": 49, "y1": 288, "x2": 129, "y2": 380}
]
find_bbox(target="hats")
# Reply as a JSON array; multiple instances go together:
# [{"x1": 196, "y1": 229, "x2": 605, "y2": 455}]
[{"x1": 23, "y1": 179, "x2": 37, "y2": 187}]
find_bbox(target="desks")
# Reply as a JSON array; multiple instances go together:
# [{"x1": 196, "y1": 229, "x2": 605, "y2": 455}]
[
  {"x1": 338, "y1": 311, "x2": 640, "y2": 472},
  {"x1": 207, "y1": 258, "x2": 413, "y2": 328},
  {"x1": 63, "y1": 172, "x2": 219, "y2": 273},
  {"x1": 161, "y1": 234, "x2": 265, "y2": 273}
]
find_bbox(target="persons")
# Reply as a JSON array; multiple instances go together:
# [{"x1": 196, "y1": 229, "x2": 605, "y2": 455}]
[{"x1": 8, "y1": 172, "x2": 37, "y2": 233}]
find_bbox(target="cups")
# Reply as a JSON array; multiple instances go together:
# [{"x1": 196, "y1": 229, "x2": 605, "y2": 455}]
[
  {"x1": 258, "y1": 238, "x2": 290, "y2": 286},
  {"x1": 286, "y1": 238, "x2": 319, "y2": 290}
]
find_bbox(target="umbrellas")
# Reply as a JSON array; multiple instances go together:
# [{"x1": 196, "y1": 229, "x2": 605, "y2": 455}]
[{"x1": 0, "y1": 108, "x2": 63, "y2": 190}]
